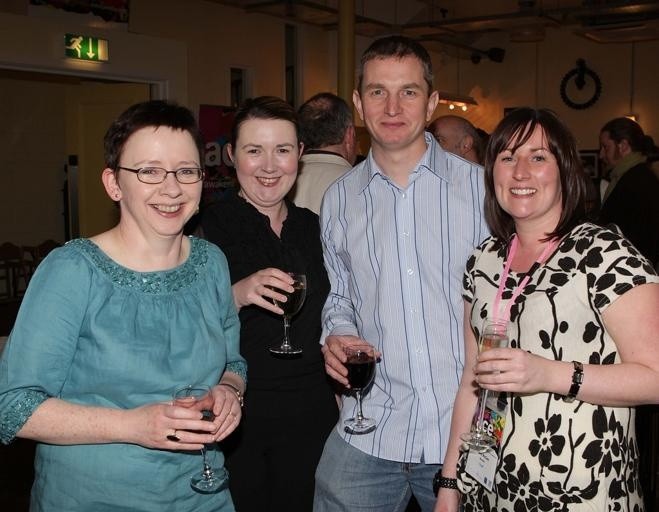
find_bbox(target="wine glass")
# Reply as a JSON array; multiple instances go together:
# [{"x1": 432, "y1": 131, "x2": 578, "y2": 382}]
[{"x1": 164, "y1": 428, "x2": 181, "y2": 442}]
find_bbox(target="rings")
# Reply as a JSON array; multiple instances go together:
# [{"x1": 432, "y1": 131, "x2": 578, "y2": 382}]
[{"x1": 164, "y1": 428, "x2": 181, "y2": 442}]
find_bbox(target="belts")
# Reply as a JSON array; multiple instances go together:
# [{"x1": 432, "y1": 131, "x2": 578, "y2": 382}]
[
  {"x1": 460, "y1": 319, "x2": 509, "y2": 449},
  {"x1": 268, "y1": 272, "x2": 307, "y2": 356},
  {"x1": 170, "y1": 385, "x2": 230, "y2": 494},
  {"x1": 343, "y1": 345, "x2": 378, "y2": 435}
]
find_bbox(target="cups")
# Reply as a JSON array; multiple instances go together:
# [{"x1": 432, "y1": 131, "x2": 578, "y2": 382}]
[{"x1": 113, "y1": 165, "x2": 206, "y2": 185}]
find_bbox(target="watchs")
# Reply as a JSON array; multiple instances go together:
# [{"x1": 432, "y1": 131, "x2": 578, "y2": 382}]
[{"x1": 433, "y1": 468, "x2": 458, "y2": 496}]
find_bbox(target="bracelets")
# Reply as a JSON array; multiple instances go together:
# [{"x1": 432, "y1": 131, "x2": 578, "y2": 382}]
[{"x1": 562, "y1": 361, "x2": 584, "y2": 403}]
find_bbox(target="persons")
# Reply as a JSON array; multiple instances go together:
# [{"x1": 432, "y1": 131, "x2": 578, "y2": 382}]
[
  {"x1": 429, "y1": 115, "x2": 489, "y2": 167},
  {"x1": 183, "y1": 91, "x2": 370, "y2": 512},
  {"x1": 312, "y1": 36, "x2": 491, "y2": 512},
  {"x1": 599, "y1": 117, "x2": 659, "y2": 270},
  {"x1": 0, "y1": 100, "x2": 248, "y2": 512},
  {"x1": 433, "y1": 108, "x2": 659, "y2": 512}
]
